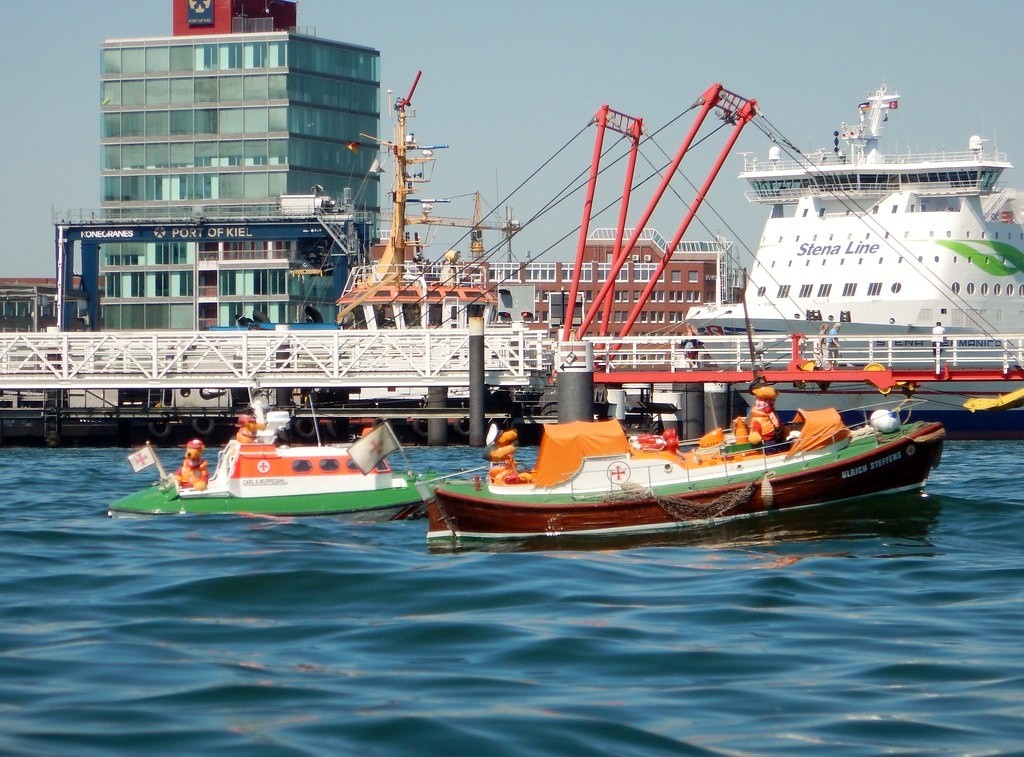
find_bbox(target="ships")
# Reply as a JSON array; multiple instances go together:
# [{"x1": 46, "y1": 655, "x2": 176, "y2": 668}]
[{"x1": 680, "y1": 84, "x2": 1024, "y2": 436}]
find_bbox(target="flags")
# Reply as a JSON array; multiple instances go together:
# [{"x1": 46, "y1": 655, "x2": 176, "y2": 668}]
[
  {"x1": 347, "y1": 142, "x2": 360, "y2": 154},
  {"x1": 858, "y1": 102, "x2": 870, "y2": 111},
  {"x1": 883, "y1": 110, "x2": 888, "y2": 122},
  {"x1": 126, "y1": 448, "x2": 154, "y2": 471},
  {"x1": 346, "y1": 425, "x2": 398, "y2": 477}
]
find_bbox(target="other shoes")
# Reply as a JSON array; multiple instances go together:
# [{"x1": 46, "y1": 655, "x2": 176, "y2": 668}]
[
  {"x1": 692, "y1": 365, "x2": 698, "y2": 368},
  {"x1": 686, "y1": 369, "x2": 693, "y2": 371}
]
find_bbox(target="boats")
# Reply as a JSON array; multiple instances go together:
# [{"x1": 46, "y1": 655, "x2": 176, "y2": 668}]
[
  {"x1": 105, "y1": 400, "x2": 446, "y2": 522},
  {"x1": 418, "y1": 376, "x2": 945, "y2": 554}
]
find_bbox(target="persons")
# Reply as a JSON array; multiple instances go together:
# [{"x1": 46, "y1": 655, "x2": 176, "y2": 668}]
[
  {"x1": 839, "y1": 149, "x2": 847, "y2": 164},
  {"x1": 932, "y1": 322, "x2": 945, "y2": 369},
  {"x1": 520, "y1": 311, "x2": 534, "y2": 321},
  {"x1": 814, "y1": 323, "x2": 841, "y2": 370},
  {"x1": 680, "y1": 325, "x2": 699, "y2": 368}
]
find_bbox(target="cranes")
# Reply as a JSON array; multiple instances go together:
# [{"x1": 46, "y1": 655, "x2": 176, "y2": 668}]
[{"x1": 371, "y1": 192, "x2": 523, "y2": 263}]
